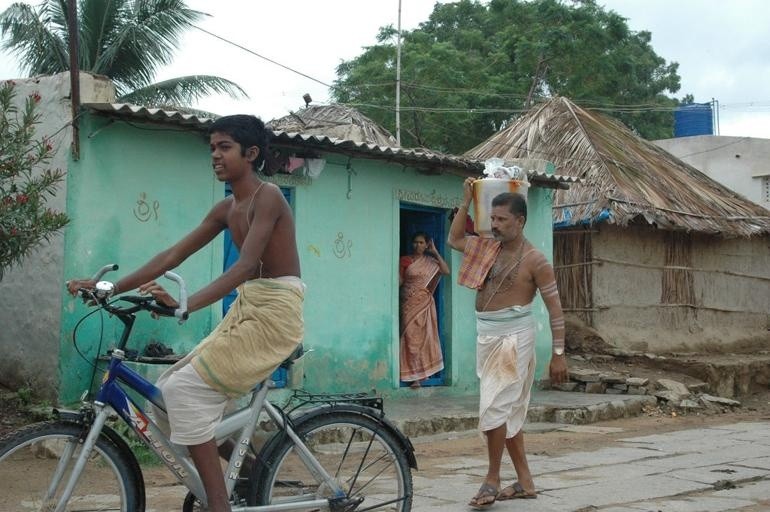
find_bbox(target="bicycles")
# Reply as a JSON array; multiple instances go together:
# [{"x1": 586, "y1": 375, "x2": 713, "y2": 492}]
[{"x1": 0, "y1": 262, "x2": 421, "y2": 512}]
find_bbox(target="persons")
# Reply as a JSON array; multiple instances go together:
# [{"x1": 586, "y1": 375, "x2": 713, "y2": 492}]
[
  {"x1": 446, "y1": 176, "x2": 569, "y2": 506},
  {"x1": 399, "y1": 231, "x2": 449, "y2": 387},
  {"x1": 66, "y1": 115, "x2": 305, "y2": 512}
]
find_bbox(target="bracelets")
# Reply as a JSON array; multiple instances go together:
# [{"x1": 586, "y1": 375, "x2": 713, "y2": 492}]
[{"x1": 552, "y1": 348, "x2": 565, "y2": 355}]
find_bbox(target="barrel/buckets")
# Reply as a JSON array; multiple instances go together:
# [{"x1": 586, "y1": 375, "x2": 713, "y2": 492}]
[
  {"x1": 472, "y1": 177, "x2": 530, "y2": 239},
  {"x1": 675, "y1": 103, "x2": 713, "y2": 137}
]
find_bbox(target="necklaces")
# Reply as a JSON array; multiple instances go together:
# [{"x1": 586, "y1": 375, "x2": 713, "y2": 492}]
[{"x1": 492, "y1": 239, "x2": 527, "y2": 294}]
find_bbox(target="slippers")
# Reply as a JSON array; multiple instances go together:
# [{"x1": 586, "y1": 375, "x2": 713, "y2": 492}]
[{"x1": 467, "y1": 482, "x2": 537, "y2": 510}]
